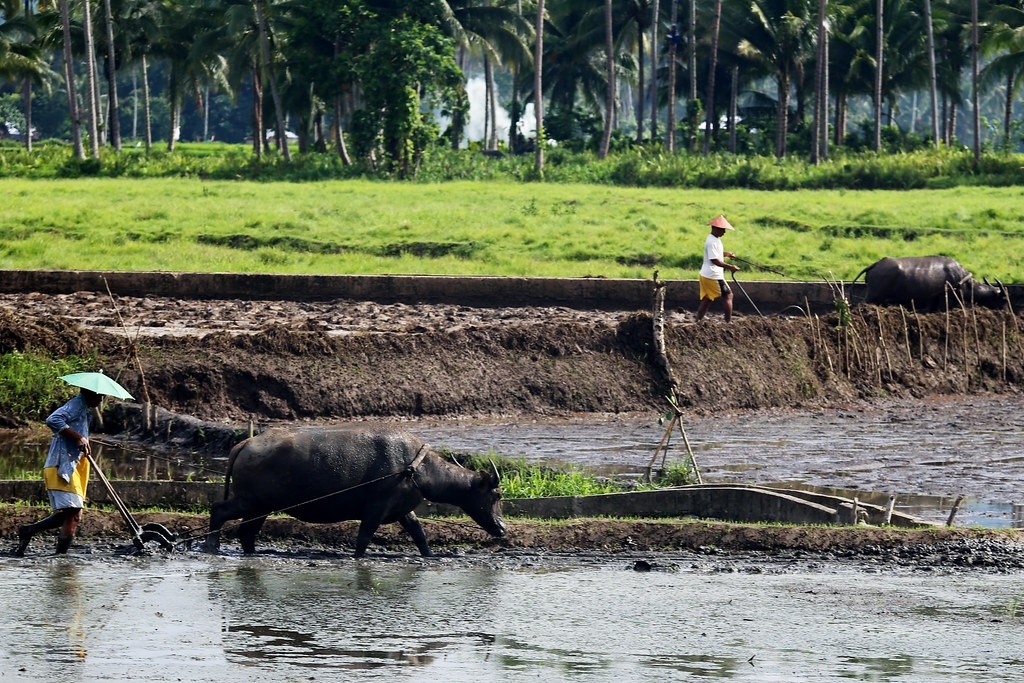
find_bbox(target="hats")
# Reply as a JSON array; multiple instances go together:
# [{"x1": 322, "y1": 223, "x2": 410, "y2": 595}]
[
  {"x1": 56, "y1": 369, "x2": 136, "y2": 401},
  {"x1": 708, "y1": 214, "x2": 735, "y2": 230}
]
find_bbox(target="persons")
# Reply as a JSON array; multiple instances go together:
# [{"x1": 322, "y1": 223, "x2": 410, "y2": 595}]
[
  {"x1": 697, "y1": 215, "x2": 739, "y2": 323},
  {"x1": 17, "y1": 387, "x2": 106, "y2": 557}
]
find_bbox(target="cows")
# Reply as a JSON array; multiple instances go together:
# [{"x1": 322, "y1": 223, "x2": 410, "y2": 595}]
[
  {"x1": 851, "y1": 255, "x2": 1007, "y2": 312},
  {"x1": 203, "y1": 430, "x2": 510, "y2": 555}
]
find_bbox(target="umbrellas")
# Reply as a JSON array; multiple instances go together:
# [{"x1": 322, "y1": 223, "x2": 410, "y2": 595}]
[{"x1": 56, "y1": 369, "x2": 136, "y2": 401}]
